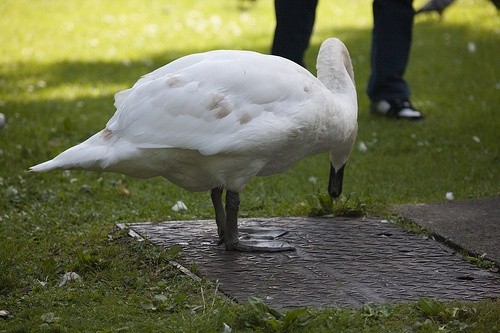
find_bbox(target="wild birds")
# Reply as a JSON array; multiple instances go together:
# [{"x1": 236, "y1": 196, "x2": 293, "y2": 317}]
[
  {"x1": 413, "y1": 0, "x2": 455, "y2": 21},
  {"x1": 26, "y1": 38, "x2": 359, "y2": 254}
]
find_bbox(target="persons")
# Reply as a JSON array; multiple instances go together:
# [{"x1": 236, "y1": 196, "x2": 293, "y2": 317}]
[{"x1": 271, "y1": 0, "x2": 425, "y2": 119}]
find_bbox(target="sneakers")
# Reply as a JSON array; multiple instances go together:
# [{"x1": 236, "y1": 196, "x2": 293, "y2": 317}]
[{"x1": 372, "y1": 95, "x2": 424, "y2": 119}]
